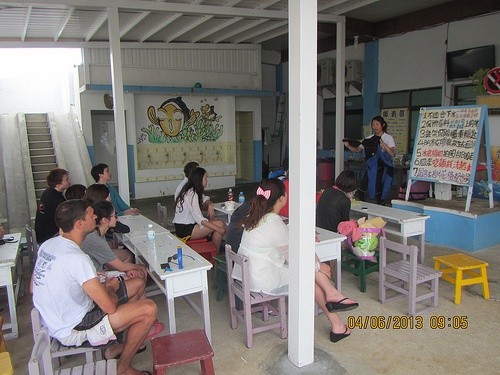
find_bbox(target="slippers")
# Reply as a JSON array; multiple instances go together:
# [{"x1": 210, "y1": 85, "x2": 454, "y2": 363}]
[
  {"x1": 104, "y1": 344, "x2": 146, "y2": 361},
  {"x1": 142, "y1": 371, "x2": 156, "y2": 375},
  {"x1": 145, "y1": 323, "x2": 164, "y2": 340}
]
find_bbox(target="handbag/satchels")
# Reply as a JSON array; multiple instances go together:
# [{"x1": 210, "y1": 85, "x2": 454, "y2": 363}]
[{"x1": 347, "y1": 228, "x2": 384, "y2": 263}]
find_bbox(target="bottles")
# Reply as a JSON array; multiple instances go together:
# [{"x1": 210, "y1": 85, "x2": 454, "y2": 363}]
[
  {"x1": 146, "y1": 223, "x2": 156, "y2": 244},
  {"x1": 228, "y1": 188, "x2": 235, "y2": 209},
  {"x1": 239, "y1": 192, "x2": 245, "y2": 206}
]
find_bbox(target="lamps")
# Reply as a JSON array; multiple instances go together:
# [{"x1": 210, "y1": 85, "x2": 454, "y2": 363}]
[{"x1": 353, "y1": 36, "x2": 360, "y2": 49}]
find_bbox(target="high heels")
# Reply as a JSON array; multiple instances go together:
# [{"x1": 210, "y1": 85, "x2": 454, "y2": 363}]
[
  {"x1": 326, "y1": 298, "x2": 359, "y2": 313},
  {"x1": 330, "y1": 327, "x2": 350, "y2": 342}
]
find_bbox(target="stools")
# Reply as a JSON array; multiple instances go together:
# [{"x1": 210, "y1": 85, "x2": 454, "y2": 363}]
[
  {"x1": 432, "y1": 253, "x2": 491, "y2": 304},
  {"x1": 184, "y1": 238, "x2": 217, "y2": 272},
  {"x1": 151, "y1": 329, "x2": 215, "y2": 375}
]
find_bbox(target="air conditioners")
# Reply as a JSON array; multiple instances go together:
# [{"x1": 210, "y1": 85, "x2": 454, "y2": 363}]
[
  {"x1": 319, "y1": 59, "x2": 336, "y2": 85},
  {"x1": 346, "y1": 60, "x2": 363, "y2": 82}
]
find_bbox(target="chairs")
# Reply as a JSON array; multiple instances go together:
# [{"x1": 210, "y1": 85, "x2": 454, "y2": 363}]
[{"x1": 0, "y1": 203, "x2": 442, "y2": 375}]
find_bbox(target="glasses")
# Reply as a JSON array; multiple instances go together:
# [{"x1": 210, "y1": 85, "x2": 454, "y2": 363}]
[
  {"x1": 0, "y1": 237, "x2": 18, "y2": 245},
  {"x1": 168, "y1": 254, "x2": 194, "y2": 266}
]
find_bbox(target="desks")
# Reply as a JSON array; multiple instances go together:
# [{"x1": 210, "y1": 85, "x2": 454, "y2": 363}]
[
  {"x1": 213, "y1": 201, "x2": 242, "y2": 226},
  {"x1": 316, "y1": 158, "x2": 434, "y2": 201},
  {"x1": 0, "y1": 233, "x2": 22, "y2": 341},
  {"x1": 351, "y1": 201, "x2": 430, "y2": 267},
  {"x1": 278, "y1": 216, "x2": 348, "y2": 295},
  {"x1": 131, "y1": 232, "x2": 213, "y2": 345},
  {"x1": 113, "y1": 214, "x2": 170, "y2": 254}
]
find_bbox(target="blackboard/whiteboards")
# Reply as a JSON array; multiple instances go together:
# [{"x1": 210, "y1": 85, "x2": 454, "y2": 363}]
[{"x1": 407, "y1": 104, "x2": 493, "y2": 187}]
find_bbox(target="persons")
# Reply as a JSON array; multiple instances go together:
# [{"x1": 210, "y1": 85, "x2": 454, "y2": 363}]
[
  {"x1": 172, "y1": 161, "x2": 227, "y2": 257},
  {"x1": 0, "y1": 226, "x2": 4, "y2": 239},
  {"x1": 341, "y1": 115, "x2": 396, "y2": 206},
  {"x1": 58, "y1": 183, "x2": 165, "y2": 339},
  {"x1": 225, "y1": 179, "x2": 360, "y2": 343},
  {"x1": 29, "y1": 199, "x2": 158, "y2": 375},
  {"x1": 90, "y1": 163, "x2": 140, "y2": 217},
  {"x1": 34, "y1": 167, "x2": 71, "y2": 247},
  {"x1": 316, "y1": 169, "x2": 357, "y2": 233},
  {"x1": 269, "y1": 157, "x2": 289, "y2": 218}
]
